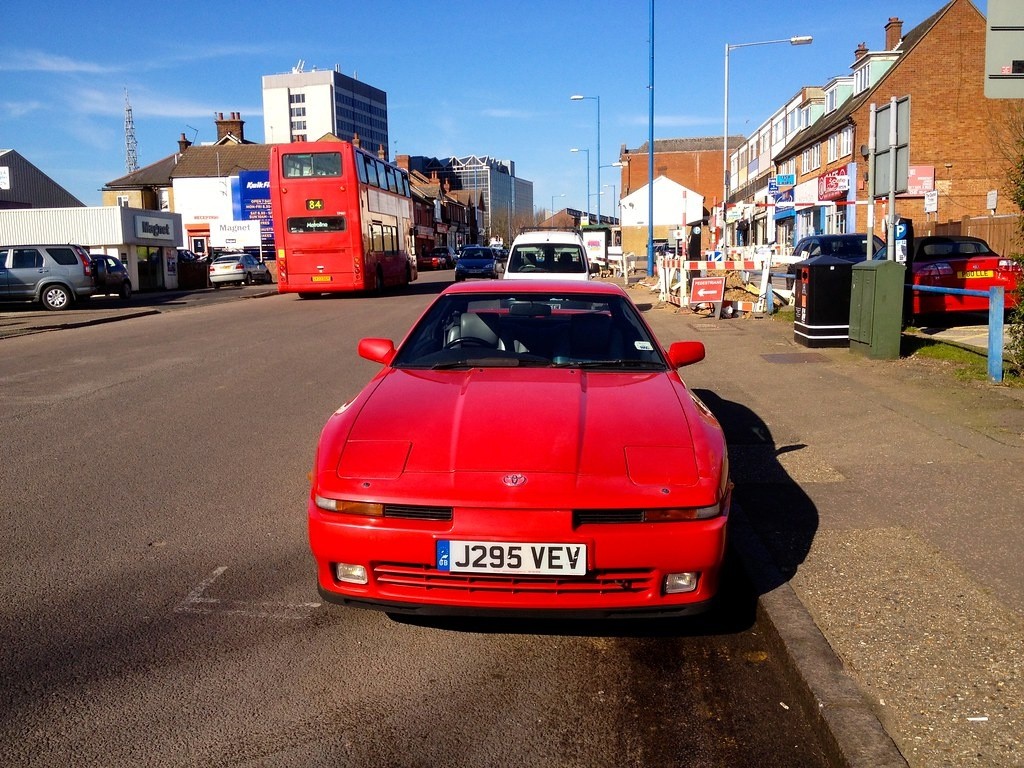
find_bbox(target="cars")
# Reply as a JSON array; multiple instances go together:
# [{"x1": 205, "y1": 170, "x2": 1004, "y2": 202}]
[
  {"x1": 308, "y1": 281, "x2": 735, "y2": 617},
  {"x1": 455, "y1": 246, "x2": 498, "y2": 281},
  {"x1": 430, "y1": 246, "x2": 457, "y2": 269},
  {"x1": 872, "y1": 234, "x2": 1024, "y2": 333},
  {"x1": 89, "y1": 255, "x2": 132, "y2": 299},
  {"x1": 492, "y1": 247, "x2": 508, "y2": 262},
  {"x1": 209, "y1": 254, "x2": 272, "y2": 290},
  {"x1": 786, "y1": 232, "x2": 886, "y2": 290}
]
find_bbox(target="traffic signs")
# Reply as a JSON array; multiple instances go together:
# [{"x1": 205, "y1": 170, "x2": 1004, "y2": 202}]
[{"x1": 690, "y1": 278, "x2": 726, "y2": 302}]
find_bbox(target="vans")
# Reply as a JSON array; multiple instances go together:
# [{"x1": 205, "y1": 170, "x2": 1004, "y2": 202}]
[
  {"x1": 503, "y1": 231, "x2": 589, "y2": 280},
  {"x1": 0, "y1": 244, "x2": 96, "y2": 311}
]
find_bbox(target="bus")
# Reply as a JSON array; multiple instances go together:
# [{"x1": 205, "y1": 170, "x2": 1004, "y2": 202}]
[{"x1": 269, "y1": 143, "x2": 418, "y2": 299}]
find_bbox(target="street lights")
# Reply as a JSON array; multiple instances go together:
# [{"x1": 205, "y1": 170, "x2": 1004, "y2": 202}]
[
  {"x1": 722, "y1": 35, "x2": 812, "y2": 262},
  {"x1": 551, "y1": 194, "x2": 566, "y2": 227},
  {"x1": 571, "y1": 96, "x2": 628, "y2": 225}
]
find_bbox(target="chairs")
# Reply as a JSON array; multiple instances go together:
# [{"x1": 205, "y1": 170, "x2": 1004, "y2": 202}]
[
  {"x1": 554, "y1": 309, "x2": 630, "y2": 363},
  {"x1": 556, "y1": 253, "x2": 577, "y2": 271},
  {"x1": 521, "y1": 252, "x2": 544, "y2": 272},
  {"x1": 464, "y1": 251, "x2": 474, "y2": 259},
  {"x1": 447, "y1": 310, "x2": 529, "y2": 362}
]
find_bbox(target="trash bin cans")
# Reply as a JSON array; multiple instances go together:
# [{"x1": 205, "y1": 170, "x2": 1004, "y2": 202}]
[{"x1": 794, "y1": 254, "x2": 856, "y2": 349}]
